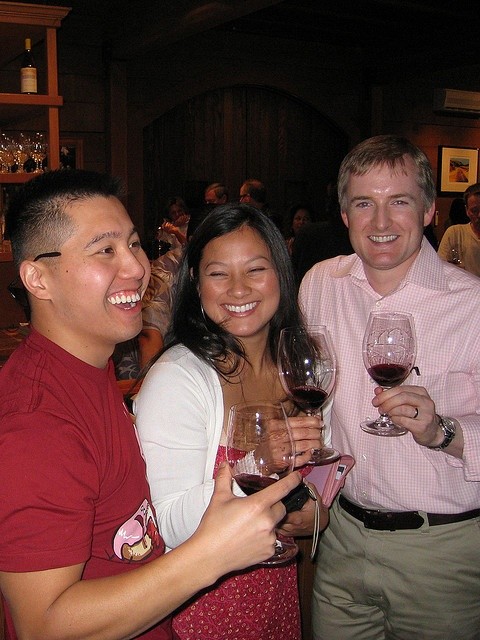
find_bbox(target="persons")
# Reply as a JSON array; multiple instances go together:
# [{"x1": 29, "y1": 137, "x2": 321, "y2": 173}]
[
  {"x1": 287, "y1": 205, "x2": 325, "y2": 255},
  {"x1": 157, "y1": 196, "x2": 191, "y2": 248},
  {"x1": 443, "y1": 197, "x2": 469, "y2": 231},
  {"x1": 202, "y1": 181, "x2": 229, "y2": 204},
  {"x1": 437, "y1": 184, "x2": 480, "y2": 277},
  {"x1": 297, "y1": 133, "x2": 479, "y2": 639},
  {"x1": 240, "y1": 178, "x2": 290, "y2": 241},
  {"x1": 135, "y1": 203, "x2": 330, "y2": 638},
  {"x1": 135, "y1": 205, "x2": 222, "y2": 394},
  {"x1": 0, "y1": 168, "x2": 303, "y2": 639}
]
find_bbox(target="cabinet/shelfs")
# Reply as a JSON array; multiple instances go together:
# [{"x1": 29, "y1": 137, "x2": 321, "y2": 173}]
[{"x1": 1, "y1": 0, "x2": 74, "y2": 357}]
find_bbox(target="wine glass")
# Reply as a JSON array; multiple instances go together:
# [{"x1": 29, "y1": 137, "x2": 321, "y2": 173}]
[
  {"x1": 446, "y1": 244, "x2": 466, "y2": 264},
  {"x1": 225, "y1": 402, "x2": 299, "y2": 566},
  {"x1": 277, "y1": 325, "x2": 341, "y2": 465},
  {"x1": 360, "y1": 311, "x2": 417, "y2": 437},
  {"x1": 0, "y1": 132, "x2": 49, "y2": 174}
]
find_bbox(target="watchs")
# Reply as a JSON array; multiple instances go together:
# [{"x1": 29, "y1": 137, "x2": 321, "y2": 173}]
[{"x1": 426, "y1": 414, "x2": 456, "y2": 451}]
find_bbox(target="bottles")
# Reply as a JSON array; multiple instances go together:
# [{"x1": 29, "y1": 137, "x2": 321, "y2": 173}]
[{"x1": 20, "y1": 38, "x2": 37, "y2": 94}]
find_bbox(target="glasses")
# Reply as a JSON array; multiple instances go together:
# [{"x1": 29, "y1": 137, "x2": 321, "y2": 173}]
[
  {"x1": 237, "y1": 193, "x2": 250, "y2": 200},
  {"x1": 7, "y1": 252, "x2": 61, "y2": 320}
]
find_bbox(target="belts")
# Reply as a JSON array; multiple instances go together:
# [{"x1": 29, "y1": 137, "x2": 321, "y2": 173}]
[{"x1": 337, "y1": 494, "x2": 480, "y2": 533}]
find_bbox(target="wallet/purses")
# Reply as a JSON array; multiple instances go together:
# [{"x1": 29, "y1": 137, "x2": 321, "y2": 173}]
[{"x1": 301, "y1": 454, "x2": 356, "y2": 507}]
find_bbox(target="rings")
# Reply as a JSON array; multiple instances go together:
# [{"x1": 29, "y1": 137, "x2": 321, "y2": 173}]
[{"x1": 414, "y1": 408, "x2": 418, "y2": 418}]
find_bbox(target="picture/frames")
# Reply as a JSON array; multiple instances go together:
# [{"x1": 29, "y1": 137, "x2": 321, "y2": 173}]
[{"x1": 435, "y1": 143, "x2": 480, "y2": 196}]
